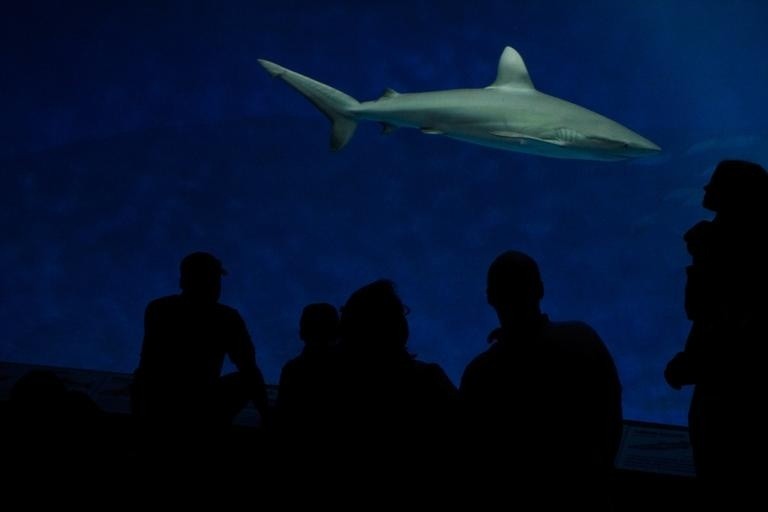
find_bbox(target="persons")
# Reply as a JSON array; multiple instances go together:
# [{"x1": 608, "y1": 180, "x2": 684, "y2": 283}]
[
  {"x1": 273, "y1": 300, "x2": 343, "y2": 512},
  {"x1": 127, "y1": 252, "x2": 273, "y2": 436},
  {"x1": 332, "y1": 277, "x2": 460, "y2": 512},
  {"x1": 659, "y1": 158, "x2": 767, "y2": 512},
  {"x1": 460, "y1": 249, "x2": 626, "y2": 512}
]
